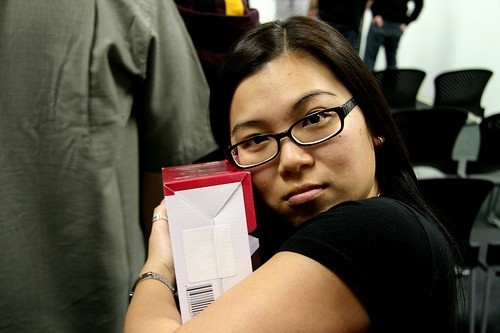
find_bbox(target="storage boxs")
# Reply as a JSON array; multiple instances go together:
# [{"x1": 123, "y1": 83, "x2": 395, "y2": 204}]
[{"x1": 162, "y1": 159, "x2": 262, "y2": 325}]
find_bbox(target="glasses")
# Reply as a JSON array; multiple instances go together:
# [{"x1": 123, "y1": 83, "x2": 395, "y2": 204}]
[{"x1": 227, "y1": 97, "x2": 358, "y2": 169}]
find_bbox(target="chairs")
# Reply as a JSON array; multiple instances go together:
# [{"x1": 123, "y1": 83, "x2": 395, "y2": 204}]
[{"x1": 369, "y1": 68, "x2": 500, "y2": 333}]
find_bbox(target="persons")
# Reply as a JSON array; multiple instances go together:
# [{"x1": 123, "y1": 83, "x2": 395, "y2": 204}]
[
  {"x1": 307, "y1": 0, "x2": 424, "y2": 73},
  {"x1": 122, "y1": 16, "x2": 458, "y2": 333},
  {"x1": 1, "y1": 0, "x2": 222, "y2": 333}
]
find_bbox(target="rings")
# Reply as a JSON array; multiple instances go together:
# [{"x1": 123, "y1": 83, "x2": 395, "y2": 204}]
[{"x1": 151, "y1": 215, "x2": 168, "y2": 223}]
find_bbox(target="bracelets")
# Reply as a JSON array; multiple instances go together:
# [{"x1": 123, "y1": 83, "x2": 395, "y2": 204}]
[{"x1": 129, "y1": 272, "x2": 178, "y2": 303}]
